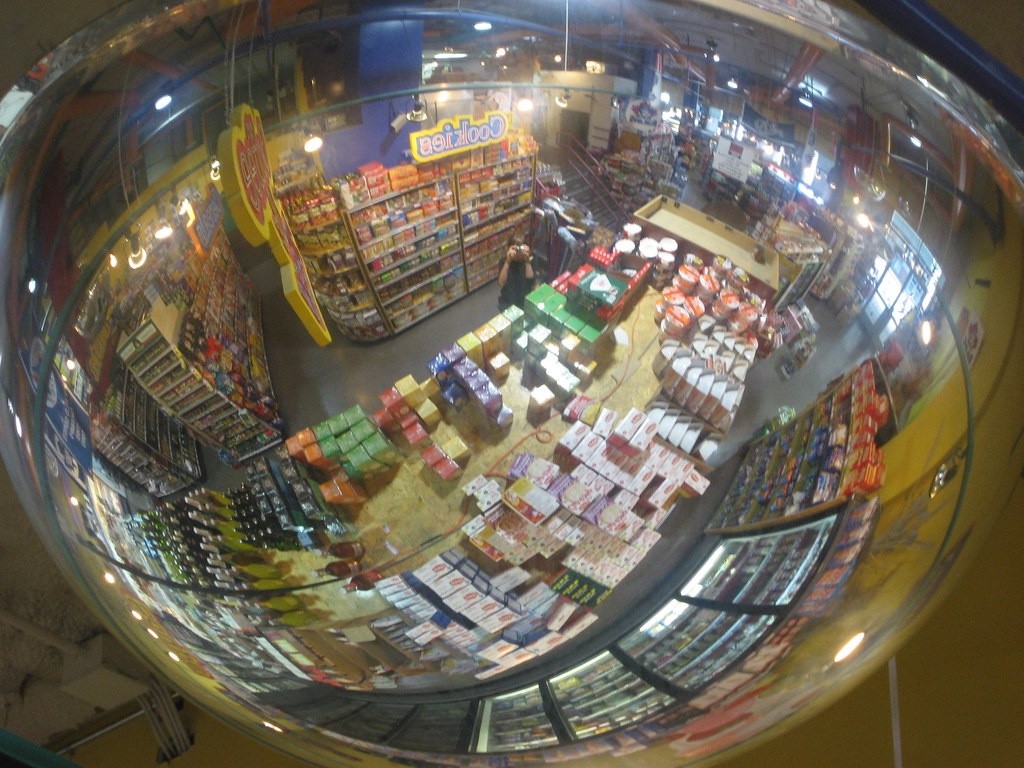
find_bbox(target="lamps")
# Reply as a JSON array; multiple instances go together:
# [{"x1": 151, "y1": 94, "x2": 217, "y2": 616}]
[
  {"x1": 727, "y1": 77, "x2": 738, "y2": 90},
  {"x1": 474, "y1": 20, "x2": 493, "y2": 31},
  {"x1": 168, "y1": 195, "x2": 188, "y2": 215},
  {"x1": 301, "y1": 128, "x2": 322, "y2": 152},
  {"x1": 124, "y1": 229, "x2": 149, "y2": 268},
  {"x1": 815, "y1": 169, "x2": 821, "y2": 180},
  {"x1": 702, "y1": 40, "x2": 720, "y2": 63},
  {"x1": 405, "y1": 94, "x2": 427, "y2": 121},
  {"x1": 208, "y1": 157, "x2": 220, "y2": 181},
  {"x1": 556, "y1": 89, "x2": 571, "y2": 108},
  {"x1": 799, "y1": 88, "x2": 814, "y2": 109},
  {"x1": 153, "y1": 200, "x2": 172, "y2": 239},
  {"x1": 907, "y1": 112, "x2": 923, "y2": 149},
  {"x1": 517, "y1": 94, "x2": 534, "y2": 111},
  {"x1": 922, "y1": 317, "x2": 935, "y2": 344}
]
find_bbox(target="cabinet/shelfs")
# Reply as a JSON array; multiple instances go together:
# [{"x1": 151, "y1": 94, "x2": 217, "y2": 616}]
[
  {"x1": 477, "y1": 514, "x2": 839, "y2": 755},
  {"x1": 680, "y1": 129, "x2": 892, "y2": 331},
  {"x1": 84, "y1": 124, "x2": 538, "y2": 498},
  {"x1": 703, "y1": 354, "x2": 897, "y2": 536}
]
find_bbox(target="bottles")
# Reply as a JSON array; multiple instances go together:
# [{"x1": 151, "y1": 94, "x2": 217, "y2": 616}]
[
  {"x1": 310, "y1": 541, "x2": 383, "y2": 597},
  {"x1": 120, "y1": 482, "x2": 276, "y2": 597},
  {"x1": 486, "y1": 519, "x2": 834, "y2": 755}
]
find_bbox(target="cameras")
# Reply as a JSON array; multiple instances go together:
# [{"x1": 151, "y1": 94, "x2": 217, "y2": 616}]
[{"x1": 514, "y1": 245, "x2": 524, "y2": 254}]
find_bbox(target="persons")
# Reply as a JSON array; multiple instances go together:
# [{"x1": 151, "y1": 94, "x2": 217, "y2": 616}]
[{"x1": 497, "y1": 233, "x2": 536, "y2": 313}]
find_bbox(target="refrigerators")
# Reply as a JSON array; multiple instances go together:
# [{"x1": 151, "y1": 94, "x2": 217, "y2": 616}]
[{"x1": 461, "y1": 509, "x2": 843, "y2": 757}]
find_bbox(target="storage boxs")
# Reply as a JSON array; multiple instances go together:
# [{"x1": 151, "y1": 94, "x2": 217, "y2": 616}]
[{"x1": 284, "y1": 245, "x2": 769, "y2": 682}]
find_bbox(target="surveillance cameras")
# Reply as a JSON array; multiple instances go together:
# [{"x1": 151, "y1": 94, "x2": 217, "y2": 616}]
[{"x1": 390, "y1": 111, "x2": 408, "y2": 135}]
